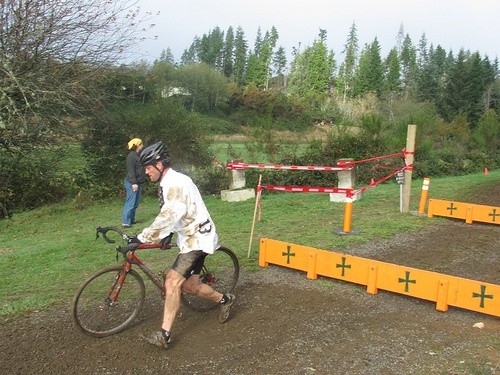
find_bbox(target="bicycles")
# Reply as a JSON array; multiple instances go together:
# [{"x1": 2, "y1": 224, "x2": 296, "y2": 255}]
[{"x1": 71, "y1": 226, "x2": 240, "y2": 339}]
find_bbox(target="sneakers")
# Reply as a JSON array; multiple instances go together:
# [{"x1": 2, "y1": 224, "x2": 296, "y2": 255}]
[
  {"x1": 140, "y1": 329, "x2": 172, "y2": 349},
  {"x1": 218, "y1": 293, "x2": 235, "y2": 323}
]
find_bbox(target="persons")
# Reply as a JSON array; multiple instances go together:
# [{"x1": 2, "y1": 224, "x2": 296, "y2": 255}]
[
  {"x1": 122, "y1": 138, "x2": 146, "y2": 228},
  {"x1": 125, "y1": 142, "x2": 236, "y2": 349}
]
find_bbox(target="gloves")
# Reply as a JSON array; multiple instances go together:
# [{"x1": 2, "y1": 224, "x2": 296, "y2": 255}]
[
  {"x1": 161, "y1": 232, "x2": 174, "y2": 250},
  {"x1": 127, "y1": 235, "x2": 142, "y2": 245}
]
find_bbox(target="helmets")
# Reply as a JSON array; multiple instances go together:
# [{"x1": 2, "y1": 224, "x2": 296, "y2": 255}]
[{"x1": 139, "y1": 141, "x2": 170, "y2": 166}]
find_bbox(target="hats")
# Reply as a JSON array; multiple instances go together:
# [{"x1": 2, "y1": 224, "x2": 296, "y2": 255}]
[{"x1": 127, "y1": 138, "x2": 142, "y2": 150}]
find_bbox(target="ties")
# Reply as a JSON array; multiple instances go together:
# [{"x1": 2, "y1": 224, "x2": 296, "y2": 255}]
[{"x1": 157, "y1": 186, "x2": 165, "y2": 211}]
